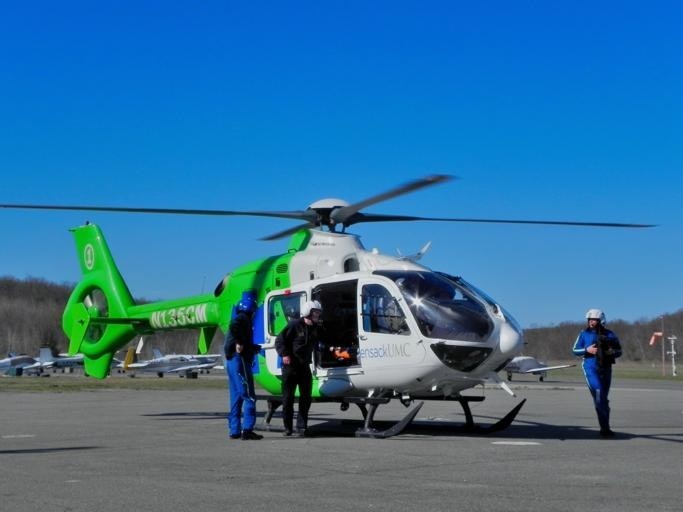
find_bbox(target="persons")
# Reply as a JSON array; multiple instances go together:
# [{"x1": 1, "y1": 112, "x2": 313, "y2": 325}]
[
  {"x1": 225, "y1": 288, "x2": 264, "y2": 440},
  {"x1": 274, "y1": 300, "x2": 336, "y2": 438},
  {"x1": 572, "y1": 309, "x2": 623, "y2": 438}
]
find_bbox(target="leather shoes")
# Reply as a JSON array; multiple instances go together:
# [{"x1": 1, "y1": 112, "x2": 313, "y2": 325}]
[
  {"x1": 297, "y1": 428, "x2": 308, "y2": 435},
  {"x1": 241, "y1": 430, "x2": 263, "y2": 440},
  {"x1": 600, "y1": 429, "x2": 615, "y2": 437},
  {"x1": 230, "y1": 434, "x2": 242, "y2": 438},
  {"x1": 282, "y1": 429, "x2": 292, "y2": 436}
]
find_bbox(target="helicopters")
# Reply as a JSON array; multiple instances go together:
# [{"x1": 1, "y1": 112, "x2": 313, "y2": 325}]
[{"x1": 0, "y1": 173, "x2": 657, "y2": 439}]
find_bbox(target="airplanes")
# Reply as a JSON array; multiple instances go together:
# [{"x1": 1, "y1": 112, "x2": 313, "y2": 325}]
[
  {"x1": 503, "y1": 355, "x2": 576, "y2": 383},
  {"x1": 9, "y1": 343, "x2": 122, "y2": 374},
  {"x1": 115, "y1": 347, "x2": 222, "y2": 378},
  {"x1": 1, "y1": 355, "x2": 43, "y2": 377}
]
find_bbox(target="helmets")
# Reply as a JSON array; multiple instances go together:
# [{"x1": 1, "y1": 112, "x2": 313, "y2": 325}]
[
  {"x1": 585, "y1": 309, "x2": 606, "y2": 325},
  {"x1": 299, "y1": 300, "x2": 322, "y2": 318},
  {"x1": 235, "y1": 298, "x2": 258, "y2": 314}
]
